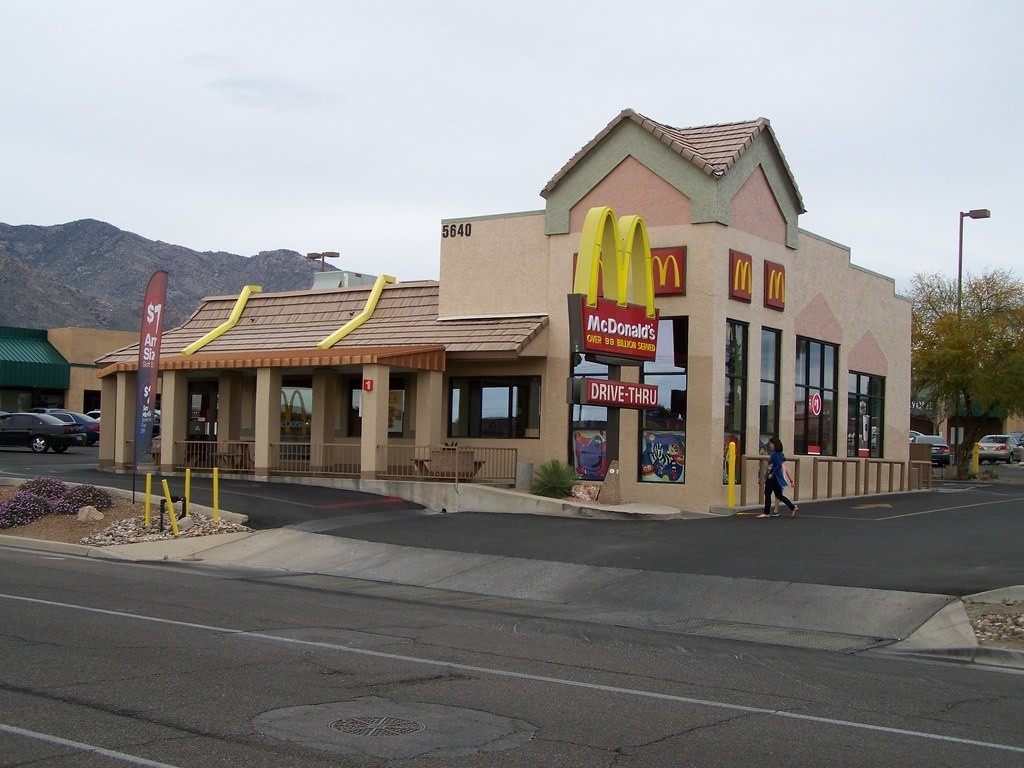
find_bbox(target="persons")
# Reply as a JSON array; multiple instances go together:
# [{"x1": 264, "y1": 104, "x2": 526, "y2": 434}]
[{"x1": 756, "y1": 437, "x2": 799, "y2": 519}]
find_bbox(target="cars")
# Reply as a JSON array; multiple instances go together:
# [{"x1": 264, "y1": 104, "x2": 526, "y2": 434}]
[
  {"x1": 25, "y1": 407, "x2": 161, "y2": 453},
  {"x1": 870, "y1": 425, "x2": 925, "y2": 451},
  {"x1": 974, "y1": 434, "x2": 1024, "y2": 465},
  {"x1": 911, "y1": 434, "x2": 951, "y2": 468},
  {"x1": 1006, "y1": 432, "x2": 1024, "y2": 446},
  {"x1": 0, "y1": 411, "x2": 88, "y2": 454}
]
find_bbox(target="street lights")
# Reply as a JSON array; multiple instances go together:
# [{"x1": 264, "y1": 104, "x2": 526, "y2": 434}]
[{"x1": 958, "y1": 208, "x2": 990, "y2": 320}]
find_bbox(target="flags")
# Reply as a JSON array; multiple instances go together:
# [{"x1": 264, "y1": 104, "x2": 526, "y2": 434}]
[{"x1": 135, "y1": 271, "x2": 169, "y2": 467}]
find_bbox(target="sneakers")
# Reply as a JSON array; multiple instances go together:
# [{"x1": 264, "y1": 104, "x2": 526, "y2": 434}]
[
  {"x1": 794, "y1": 504, "x2": 798, "y2": 507},
  {"x1": 769, "y1": 512, "x2": 780, "y2": 517}
]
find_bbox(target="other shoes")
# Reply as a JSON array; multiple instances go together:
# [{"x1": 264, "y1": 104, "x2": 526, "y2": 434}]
[
  {"x1": 756, "y1": 513, "x2": 771, "y2": 518},
  {"x1": 792, "y1": 507, "x2": 799, "y2": 519}
]
